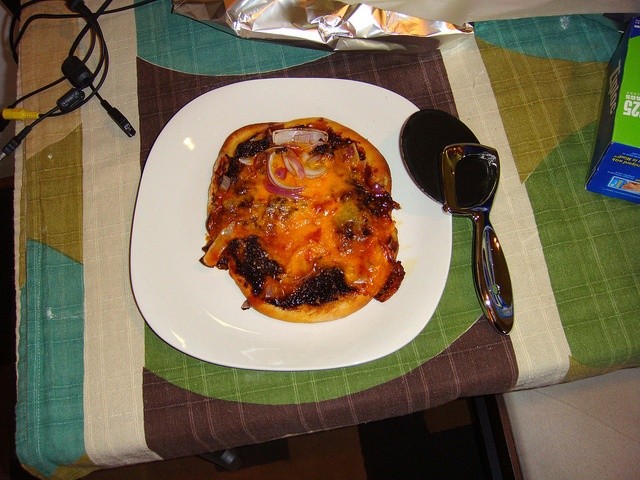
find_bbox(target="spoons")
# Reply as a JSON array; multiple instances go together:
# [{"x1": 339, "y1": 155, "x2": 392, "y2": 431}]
[{"x1": 440, "y1": 142, "x2": 515, "y2": 336}]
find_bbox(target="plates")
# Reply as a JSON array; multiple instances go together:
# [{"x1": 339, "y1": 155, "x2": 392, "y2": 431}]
[{"x1": 128, "y1": 78, "x2": 452, "y2": 372}]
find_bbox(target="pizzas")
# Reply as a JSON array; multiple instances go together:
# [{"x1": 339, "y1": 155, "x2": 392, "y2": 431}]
[{"x1": 200, "y1": 116, "x2": 407, "y2": 324}]
